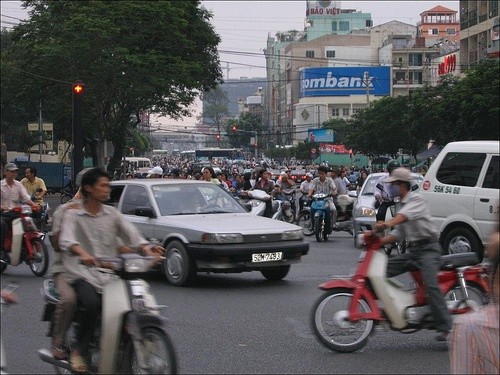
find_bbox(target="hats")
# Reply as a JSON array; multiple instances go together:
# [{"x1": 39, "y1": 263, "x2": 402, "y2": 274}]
[
  {"x1": 306, "y1": 173, "x2": 313, "y2": 178},
  {"x1": 384, "y1": 168, "x2": 411, "y2": 182},
  {"x1": 5, "y1": 163, "x2": 19, "y2": 171}
]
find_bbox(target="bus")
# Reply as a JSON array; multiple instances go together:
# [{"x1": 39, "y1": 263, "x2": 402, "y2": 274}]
[
  {"x1": 195, "y1": 149, "x2": 245, "y2": 164},
  {"x1": 179, "y1": 151, "x2": 195, "y2": 160},
  {"x1": 122, "y1": 157, "x2": 154, "y2": 178},
  {"x1": 153, "y1": 149, "x2": 168, "y2": 160}
]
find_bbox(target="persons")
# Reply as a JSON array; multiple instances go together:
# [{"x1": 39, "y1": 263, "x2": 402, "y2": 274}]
[
  {"x1": 331, "y1": 165, "x2": 372, "y2": 197},
  {"x1": 298, "y1": 172, "x2": 314, "y2": 210},
  {"x1": 20, "y1": 166, "x2": 47, "y2": 218},
  {"x1": 376, "y1": 167, "x2": 452, "y2": 341},
  {"x1": 275, "y1": 170, "x2": 297, "y2": 213},
  {"x1": 252, "y1": 167, "x2": 276, "y2": 217},
  {"x1": 449, "y1": 262, "x2": 500, "y2": 375},
  {"x1": 0, "y1": 163, "x2": 35, "y2": 259},
  {"x1": 308, "y1": 166, "x2": 337, "y2": 234},
  {"x1": 148, "y1": 167, "x2": 163, "y2": 178},
  {"x1": 374, "y1": 160, "x2": 400, "y2": 222},
  {"x1": 47, "y1": 167, "x2": 166, "y2": 372},
  {"x1": 152, "y1": 155, "x2": 329, "y2": 191}
]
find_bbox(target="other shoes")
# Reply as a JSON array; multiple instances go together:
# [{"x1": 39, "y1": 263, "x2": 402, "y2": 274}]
[
  {"x1": 324, "y1": 228, "x2": 332, "y2": 234},
  {"x1": 436, "y1": 333, "x2": 450, "y2": 340},
  {"x1": 50, "y1": 340, "x2": 70, "y2": 360}
]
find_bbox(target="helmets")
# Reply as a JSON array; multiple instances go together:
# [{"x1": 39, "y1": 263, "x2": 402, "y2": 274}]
[{"x1": 387, "y1": 159, "x2": 400, "y2": 173}]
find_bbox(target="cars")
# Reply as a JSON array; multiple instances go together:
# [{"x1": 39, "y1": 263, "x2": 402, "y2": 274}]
[
  {"x1": 347, "y1": 173, "x2": 424, "y2": 247},
  {"x1": 104, "y1": 179, "x2": 311, "y2": 287},
  {"x1": 290, "y1": 170, "x2": 306, "y2": 183}
]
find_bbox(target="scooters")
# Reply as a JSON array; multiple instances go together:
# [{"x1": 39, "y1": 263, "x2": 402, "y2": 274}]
[
  {"x1": 18, "y1": 188, "x2": 51, "y2": 241},
  {"x1": 234, "y1": 169, "x2": 359, "y2": 241},
  {"x1": 0, "y1": 204, "x2": 49, "y2": 276}
]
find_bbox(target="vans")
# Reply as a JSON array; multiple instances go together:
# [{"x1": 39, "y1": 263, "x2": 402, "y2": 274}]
[{"x1": 419, "y1": 139, "x2": 500, "y2": 263}]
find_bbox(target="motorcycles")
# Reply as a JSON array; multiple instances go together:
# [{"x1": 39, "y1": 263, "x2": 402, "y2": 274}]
[
  {"x1": 310, "y1": 223, "x2": 490, "y2": 353},
  {"x1": 60, "y1": 188, "x2": 77, "y2": 204},
  {"x1": 37, "y1": 237, "x2": 180, "y2": 375},
  {"x1": 374, "y1": 184, "x2": 420, "y2": 255}
]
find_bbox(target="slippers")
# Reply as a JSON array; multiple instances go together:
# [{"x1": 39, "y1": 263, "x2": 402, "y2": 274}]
[{"x1": 69, "y1": 351, "x2": 88, "y2": 373}]
